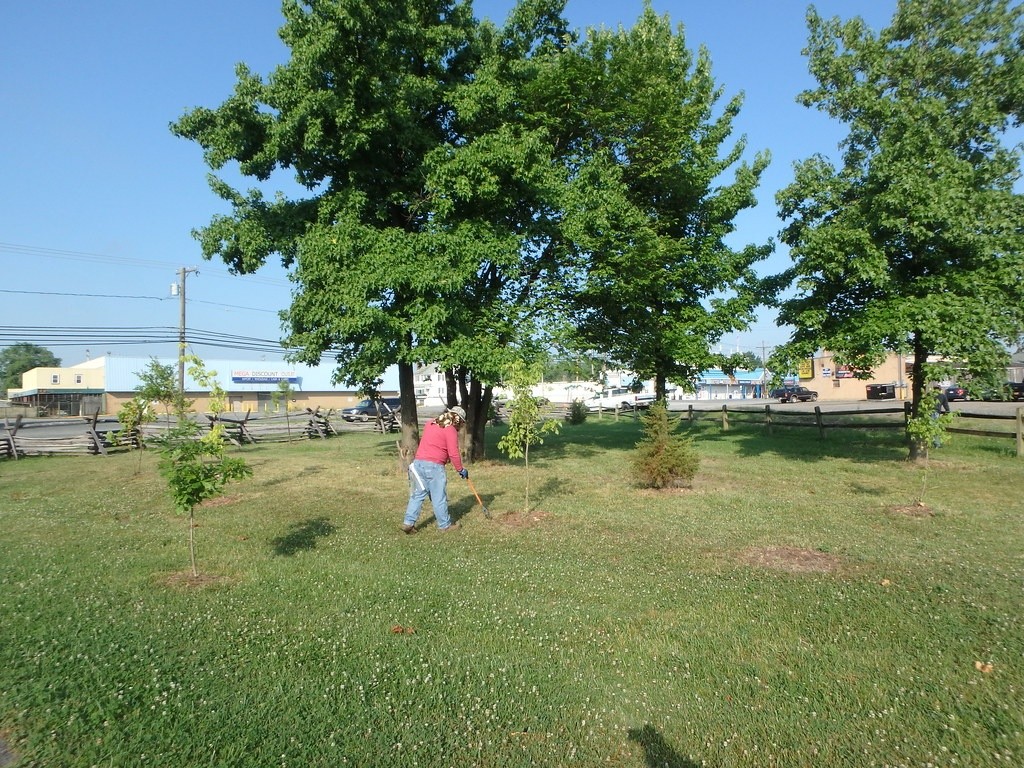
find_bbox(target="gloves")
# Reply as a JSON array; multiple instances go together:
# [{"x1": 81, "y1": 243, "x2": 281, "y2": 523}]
[{"x1": 459, "y1": 468, "x2": 468, "y2": 479}]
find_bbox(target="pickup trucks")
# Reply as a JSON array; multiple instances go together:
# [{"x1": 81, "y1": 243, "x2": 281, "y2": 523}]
[{"x1": 584, "y1": 387, "x2": 655, "y2": 411}]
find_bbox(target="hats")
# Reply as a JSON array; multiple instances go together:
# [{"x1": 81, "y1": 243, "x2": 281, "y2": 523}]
[{"x1": 447, "y1": 406, "x2": 467, "y2": 423}]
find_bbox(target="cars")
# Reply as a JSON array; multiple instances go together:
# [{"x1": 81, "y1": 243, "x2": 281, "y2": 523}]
[
  {"x1": 1004, "y1": 382, "x2": 1024, "y2": 400},
  {"x1": 341, "y1": 398, "x2": 400, "y2": 422},
  {"x1": 946, "y1": 383, "x2": 989, "y2": 401},
  {"x1": 772, "y1": 387, "x2": 818, "y2": 403}
]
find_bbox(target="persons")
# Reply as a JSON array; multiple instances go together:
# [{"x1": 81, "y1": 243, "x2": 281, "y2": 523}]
[
  {"x1": 927, "y1": 385, "x2": 950, "y2": 449},
  {"x1": 400, "y1": 406, "x2": 468, "y2": 534}
]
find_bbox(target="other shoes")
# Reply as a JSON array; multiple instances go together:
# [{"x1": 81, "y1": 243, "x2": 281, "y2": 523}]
[
  {"x1": 400, "y1": 524, "x2": 418, "y2": 533},
  {"x1": 445, "y1": 524, "x2": 462, "y2": 531}
]
